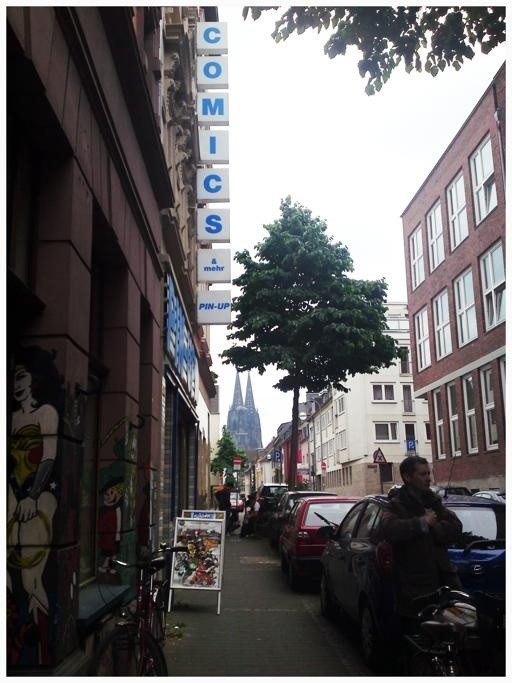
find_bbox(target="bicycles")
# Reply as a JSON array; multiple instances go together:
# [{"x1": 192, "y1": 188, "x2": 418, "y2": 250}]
[{"x1": 406, "y1": 585, "x2": 482, "y2": 677}]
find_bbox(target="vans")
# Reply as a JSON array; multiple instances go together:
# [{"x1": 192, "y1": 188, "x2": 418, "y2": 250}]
[{"x1": 270, "y1": 490, "x2": 335, "y2": 551}]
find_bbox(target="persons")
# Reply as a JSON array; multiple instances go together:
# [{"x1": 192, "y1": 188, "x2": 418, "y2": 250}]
[
  {"x1": 7, "y1": 343, "x2": 81, "y2": 626},
  {"x1": 215, "y1": 483, "x2": 260, "y2": 538},
  {"x1": 381, "y1": 455, "x2": 462, "y2": 636}
]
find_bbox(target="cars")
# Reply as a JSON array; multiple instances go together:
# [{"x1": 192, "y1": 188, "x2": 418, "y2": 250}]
[
  {"x1": 98, "y1": 545, "x2": 186, "y2": 676},
  {"x1": 320, "y1": 492, "x2": 505, "y2": 661},
  {"x1": 230, "y1": 492, "x2": 244, "y2": 513},
  {"x1": 472, "y1": 490, "x2": 505, "y2": 503},
  {"x1": 280, "y1": 496, "x2": 363, "y2": 584},
  {"x1": 431, "y1": 486, "x2": 470, "y2": 497},
  {"x1": 255, "y1": 483, "x2": 288, "y2": 510}
]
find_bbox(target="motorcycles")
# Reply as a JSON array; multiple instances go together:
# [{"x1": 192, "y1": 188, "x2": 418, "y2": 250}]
[
  {"x1": 241, "y1": 513, "x2": 256, "y2": 538},
  {"x1": 224, "y1": 509, "x2": 240, "y2": 535}
]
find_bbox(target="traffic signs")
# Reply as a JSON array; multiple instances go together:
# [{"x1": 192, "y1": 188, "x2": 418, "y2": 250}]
[{"x1": 373, "y1": 448, "x2": 387, "y2": 463}]
